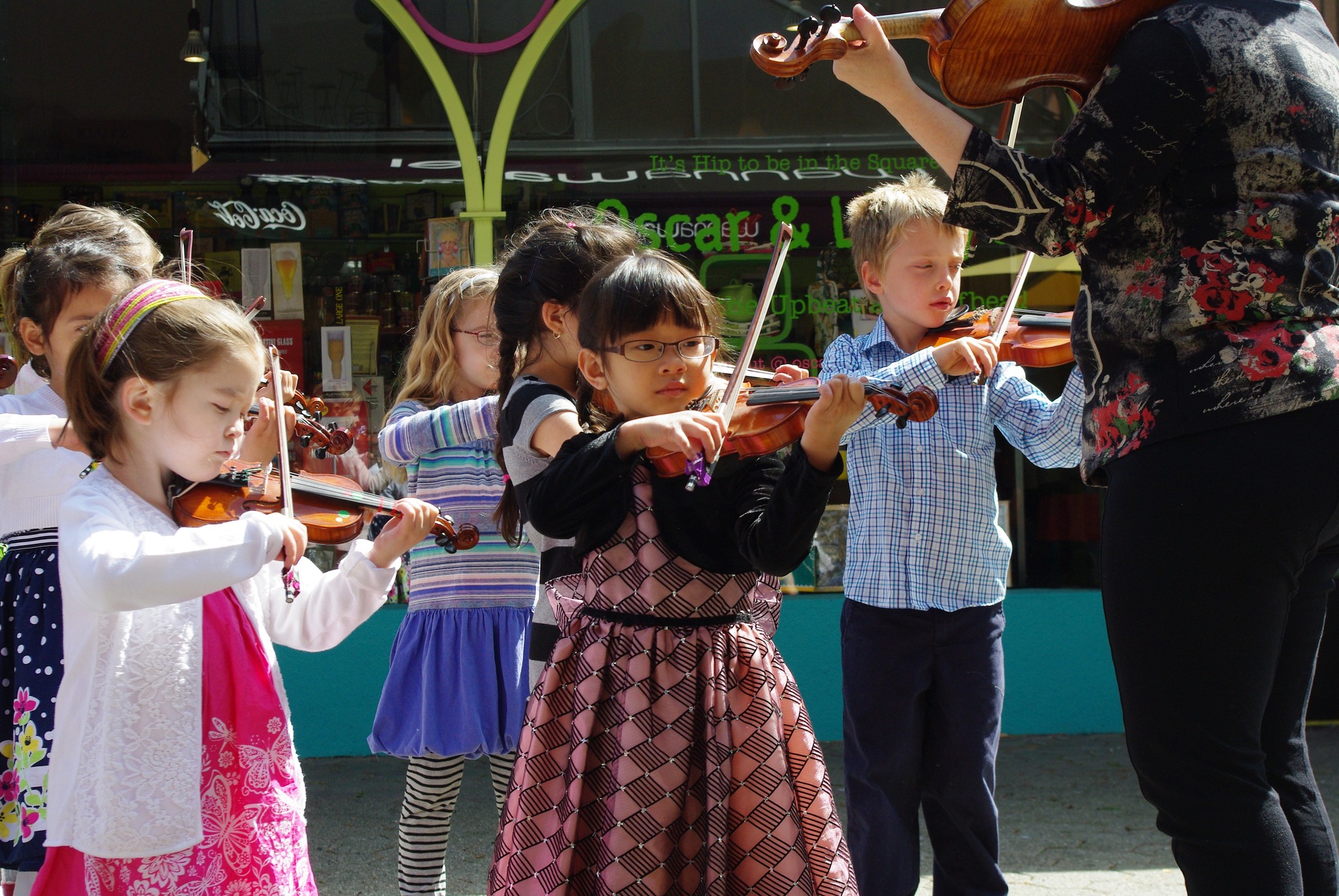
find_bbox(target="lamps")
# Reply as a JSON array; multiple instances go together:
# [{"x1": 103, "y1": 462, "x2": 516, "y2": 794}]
[{"x1": 179, "y1": 0, "x2": 210, "y2": 63}]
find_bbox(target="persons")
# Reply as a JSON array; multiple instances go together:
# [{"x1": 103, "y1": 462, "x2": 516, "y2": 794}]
[
  {"x1": 832, "y1": 0, "x2": 1339, "y2": 896},
  {"x1": 816, "y1": 169, "x2": 1087, "y2": 896},
  {"x1": 368, "y1": 268, "x2": 545, "y2": 896},
  {"x1": 491, "y1": 252, "x2": 868, "y2": 896},
  {"x1": 493, "y1": 207, "x2": 811, "y2": 689},
  {"x1": 0, "y1": 203, "x2": 438, "y2": 896}
]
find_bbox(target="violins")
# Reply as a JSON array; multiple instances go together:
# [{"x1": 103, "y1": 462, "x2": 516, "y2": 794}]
[
  {"x1": 749, "y1": 0, "x2": 1180, "y2": 111},
  {"x1": 579, "y1": 361, "x2": 785, "y2": 414},
  {"x1": 0, "y1": 354, "x2": 18, "y2": 390},
  {"x1": 644, "y1": 376, "x2": 941, "y2": 479},
  {"x1": 242, "y1": 401, "x2": 353, "y2": 461},
  {"x1": 916, "y1": 307, "x2": 1075, "y2": 368},
  {"x1": 165, "y1": 457, "x2": 479, "y2": 554},
  {"x1": 255, "y1": 374, "x2": 325, "y2": 417}
]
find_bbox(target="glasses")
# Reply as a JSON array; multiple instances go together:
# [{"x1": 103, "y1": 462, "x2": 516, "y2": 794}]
[
  {"x1": 450, "y1": 330, "x2": 502, "y2": 346},
  {"x1": 594, "y1": 335, "x2": 719, "y2": 362}
]
